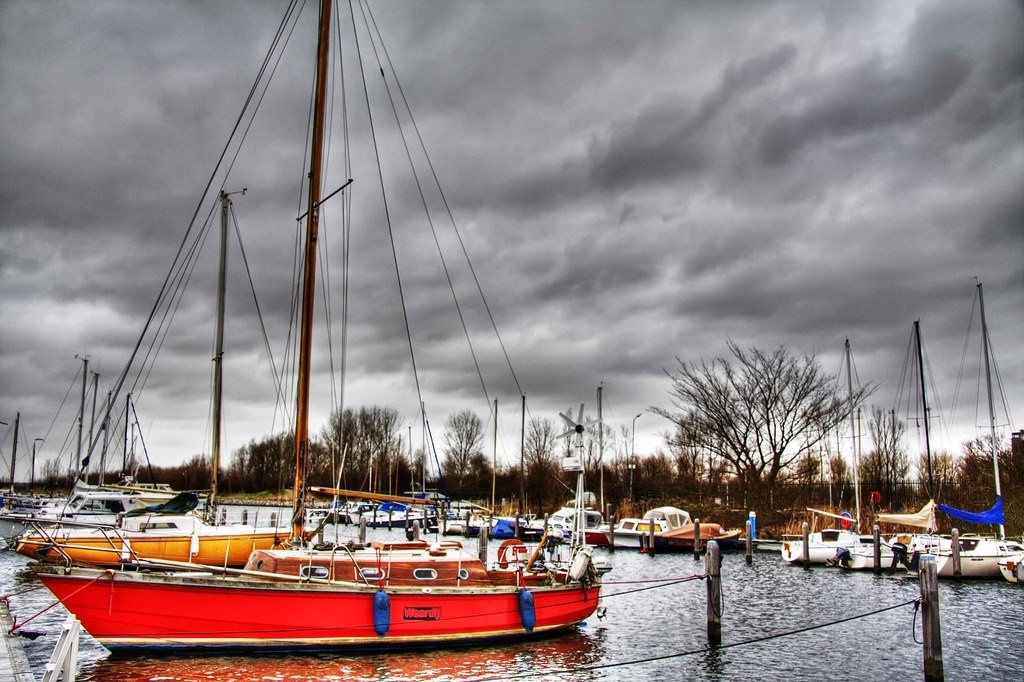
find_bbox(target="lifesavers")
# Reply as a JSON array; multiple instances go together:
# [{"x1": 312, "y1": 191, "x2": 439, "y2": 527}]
[
  {"x1": 496, "y1": 539, "x2": 528, "y2": 569},
  {"x1": 867, "y1": 491, "x2": 881, "y2": 506},
  {"x1": 840, "y1": 516, "x2": 851, "y2": 529}
]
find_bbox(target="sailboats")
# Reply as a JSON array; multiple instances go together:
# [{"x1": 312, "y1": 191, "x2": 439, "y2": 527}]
[
  {"x1": 0, "y1": 161, "x2": 1024, "y2": 587},
  {"x1": 17, "y1": 1, "x2": 617, "y2": 659}
]
